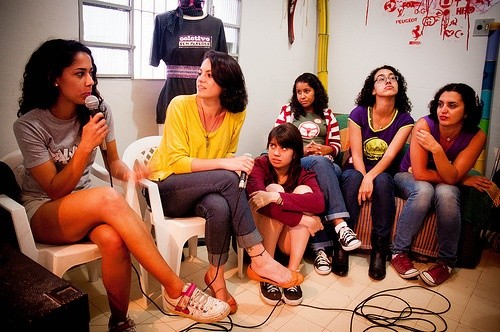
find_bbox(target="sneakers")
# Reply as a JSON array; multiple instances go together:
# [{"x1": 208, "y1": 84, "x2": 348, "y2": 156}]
[
  {"x1": 164, "y1": 282, "x2": 231, "y2": 324},
  {"x1": 421, "y1": 262, "x2": 453, "y2": 286},
  {"x1": 335, "y1": 221, "x2": 361, "y2": 251},
  {"x1": 314, "y1": 249, "x2": 332, "y2": 275},
  {"x1": 390, "y1": 253, "x2": 420, "y2": 278},
  {"x1": 108, "y1": 316, "x2": 137, "y2": 332},
  {"x1": 259, "y1": 281, "x2": 282, "y2": 306},
  {"x1": 281, "y1": 285, "x2": 304, "y2": 306}
]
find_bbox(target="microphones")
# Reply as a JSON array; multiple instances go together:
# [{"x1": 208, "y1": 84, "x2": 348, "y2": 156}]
[
  {"x1": 85, "y1": 94, "x2": 107, "y2": 156},
  {"x1": 238, "y1": 152, "x2": 253, "y2": 192}
]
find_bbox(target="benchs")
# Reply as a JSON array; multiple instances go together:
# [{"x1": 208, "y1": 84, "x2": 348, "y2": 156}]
[{"x1": 329, "y1": 113, "x2": 482, "y2": 272}]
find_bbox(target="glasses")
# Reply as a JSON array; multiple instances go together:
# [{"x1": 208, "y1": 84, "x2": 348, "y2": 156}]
[{"x1": 373, "y1": 75, "x2": 399, "y2": 84}]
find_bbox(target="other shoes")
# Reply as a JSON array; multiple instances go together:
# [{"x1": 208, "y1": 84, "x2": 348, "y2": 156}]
[
  {"x1": 246, "y1": 262, "x2": 304, "y2": 288},
  {"x1": 204, "y1": 273, "x2": 237, "y2": 314}
]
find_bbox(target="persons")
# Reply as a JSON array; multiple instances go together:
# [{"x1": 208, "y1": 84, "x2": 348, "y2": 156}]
[
  {"x1": 390, "y1": 83, "x2": 491, "y2": 286},
  {"x1": 13, "y1": 39, "x2": 231, "y2": 332},
  {"x1": 143, "y1": 49, "x2": 305, "y2": 315},
  {"x1": 332, "y1": 65, "x2": 415, "y2": 280},
  {"x1": 246, "y1": 123, "x2": 324, "y2": 305},
  {"x1": 275, "y1": 73, "x2": 362, "y2": 274}
]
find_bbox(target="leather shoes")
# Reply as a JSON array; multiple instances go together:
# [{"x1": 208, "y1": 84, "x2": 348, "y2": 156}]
[
  {"x1": 332, "y1": 250, "x2": 349, "y2": 275},
  {"x1": 368, "y1": 247, "x2": 386, "y2": 280}
]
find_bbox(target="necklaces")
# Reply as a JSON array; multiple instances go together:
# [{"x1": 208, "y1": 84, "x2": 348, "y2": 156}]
[
  {"x1": 200, "y1": 100, "x2": 224, "y2": 147},
  {"x1": 446, "y1": 137, "x2": 453, "y2": 142},
  {"x1": 380, "y1": 111, "x2": 395, "y2": 129}
]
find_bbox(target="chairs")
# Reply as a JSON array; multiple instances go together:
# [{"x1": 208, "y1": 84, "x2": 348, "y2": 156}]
[
  {"x1": 122, "y1": 137, "x2": 246, "y2": 311},
  {"x1": 0, "y1": 148, "x2": 151, "y2": 310}
]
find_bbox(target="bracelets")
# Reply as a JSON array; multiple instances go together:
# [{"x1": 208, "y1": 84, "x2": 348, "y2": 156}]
[{"x1": 277, "y1": 198, "x2": 282, "y2": 205}]
